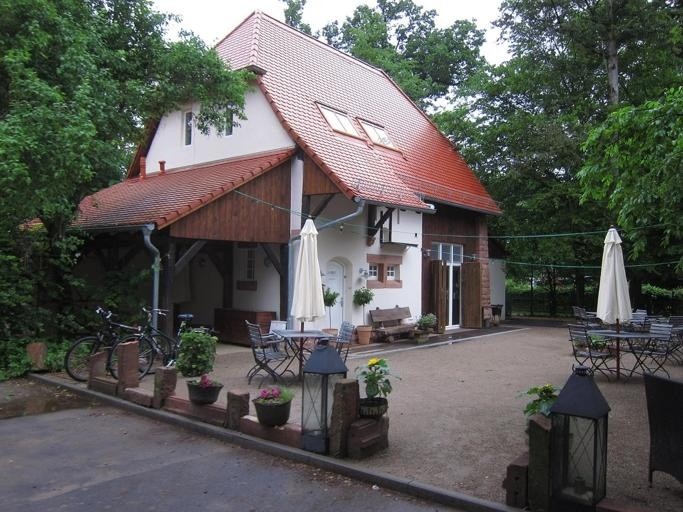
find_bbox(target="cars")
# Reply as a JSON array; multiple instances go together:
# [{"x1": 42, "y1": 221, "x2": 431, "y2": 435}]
[{"x1": 517, "y1": 273, "x2": 595, "y2": 289}]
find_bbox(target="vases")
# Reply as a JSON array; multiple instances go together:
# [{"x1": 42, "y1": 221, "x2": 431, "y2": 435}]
[
  {"x1": 253, "y1": 398, "x2": 291, "y2": 427},
  {"x1": 360, "y1": 398, "x2": 388, "y2": 417},
  {"x1": 186, "y1": 380, "x2": 223, "y2": 404}
]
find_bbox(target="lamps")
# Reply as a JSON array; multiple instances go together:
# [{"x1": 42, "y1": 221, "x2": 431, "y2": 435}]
[
  {"x1": 551, "y1": 366, "x2": 611, "y2": 505},
  {"x1": 300, "y1": 346, "x2": 348, "y2": 455}
]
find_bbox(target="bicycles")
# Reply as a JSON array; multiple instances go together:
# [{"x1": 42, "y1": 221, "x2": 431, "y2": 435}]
[
  {"x1": 66, "y1": 307, "x2": 172, "y2": 382},
  {"x1": 106, "y1": 305, "x2": 221, "y2": 383}
]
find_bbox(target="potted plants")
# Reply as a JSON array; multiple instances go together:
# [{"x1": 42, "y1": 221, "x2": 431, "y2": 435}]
[
  {"x1": 353, "y1": 288, "x2": 373, "y2": 345},
  {"x1": 323, "y1": 288, "x2": 338, "y2": 347}
]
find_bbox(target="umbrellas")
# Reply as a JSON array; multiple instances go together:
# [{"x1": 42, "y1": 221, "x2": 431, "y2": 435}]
[
  {"x1": 595, "y1": 224, "x2": 635, "y2": 383},
  {"x1": 289, "y1": 212, "x2": 326, "y2": 380}
]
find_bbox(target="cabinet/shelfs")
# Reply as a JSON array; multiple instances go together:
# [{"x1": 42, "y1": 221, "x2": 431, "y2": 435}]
[{"x1": 214, "y1": 308, "x2": 276, "y2": 346}]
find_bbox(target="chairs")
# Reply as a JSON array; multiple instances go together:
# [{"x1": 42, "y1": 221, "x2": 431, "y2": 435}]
[
  {"x1": 567, "y1": 307, "x2": 683, "y2": 384},
  {"x1": 244, "y1": 320, "x2": 355, "y2": 389}
]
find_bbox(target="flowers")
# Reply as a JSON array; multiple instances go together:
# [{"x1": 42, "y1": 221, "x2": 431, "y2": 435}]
[
  {"x1": 355, "y1": 358, "x2": 400, "y2": 400},
  {"x1": 259, "y1": 387, "x2": 280, "y2": 401},
  {"x1": 196, "y1": 375, "x2": 216, "y2": 388}
]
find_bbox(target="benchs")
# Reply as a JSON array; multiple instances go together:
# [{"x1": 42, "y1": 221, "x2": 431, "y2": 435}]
[{"x1": 369, "y1": 306, "x2": 417, "y2": 341}]
